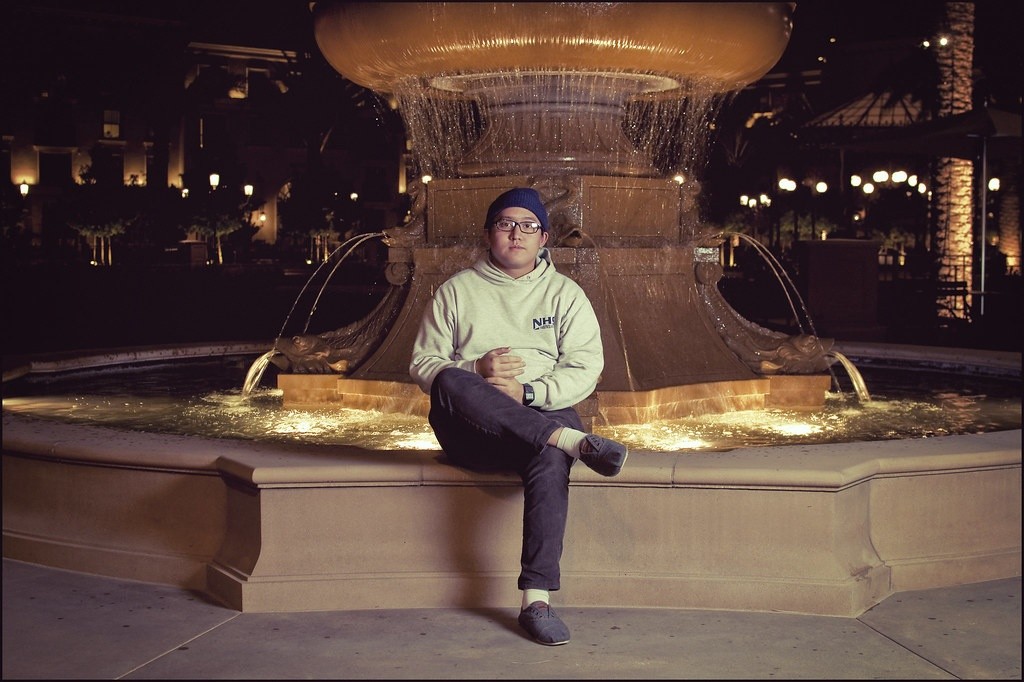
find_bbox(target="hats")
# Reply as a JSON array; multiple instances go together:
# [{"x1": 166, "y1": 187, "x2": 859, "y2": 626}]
[{"x1": 481, "y1": 187, "x2": 548, "y2": 232}]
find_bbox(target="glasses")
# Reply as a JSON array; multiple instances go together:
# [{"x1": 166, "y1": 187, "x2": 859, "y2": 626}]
[{"x1": 489, "y1": 218, "x2": 542, "y2": 234}]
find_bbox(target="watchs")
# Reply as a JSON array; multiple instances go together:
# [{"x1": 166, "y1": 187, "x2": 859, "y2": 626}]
[{"x1": 523, "y1": 383, "x2": 535, "y2": 405}]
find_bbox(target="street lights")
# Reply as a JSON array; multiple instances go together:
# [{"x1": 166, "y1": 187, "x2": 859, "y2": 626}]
[
  {"x1": 816, "y1": 181, "x2": 827, "y2": 235},
  {"x1": 987, "y1": 178, "x2": 1000, "y2": 231},
  {"x1": 774, "y1": 176, "x2": 801, "y2": 248},
  {"x1": 210, "y1": 173, "x2": 219, "y2": 262},
  {"x1": 848, "y1": 170, "x2": 926, "y2": 238},
  {"x1": 741, "y1": 194, "x2": 773, "y2": 249}
]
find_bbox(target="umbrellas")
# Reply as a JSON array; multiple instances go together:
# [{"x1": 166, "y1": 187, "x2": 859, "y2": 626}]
[{"x1": 836, "y1": 99, "x2": 1024, "y2": 315}]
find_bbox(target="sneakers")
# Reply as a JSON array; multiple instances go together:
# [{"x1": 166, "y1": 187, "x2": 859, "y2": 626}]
[
  {"x1": 578, "y1": 434, "x2": 628, "y2": 477},
  {"x1": 518, "y1": 601, "x2": 570, "y2": 645}
]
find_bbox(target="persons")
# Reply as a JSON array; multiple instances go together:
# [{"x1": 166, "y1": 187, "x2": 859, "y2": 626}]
[{"x1": 408, "y1": 187, "x2": 628, "y2": 644}]
